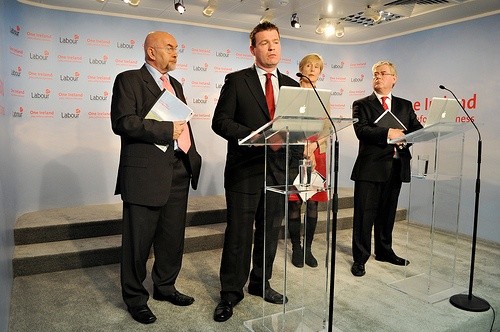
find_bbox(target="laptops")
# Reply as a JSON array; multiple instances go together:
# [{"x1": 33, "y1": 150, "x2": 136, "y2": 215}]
[
  {"x1": 400, "y1": 97, "x2": 462, "y2": 143},
  {"x1": 251, "y1": 86, "x2": 331, "y2": 144}
]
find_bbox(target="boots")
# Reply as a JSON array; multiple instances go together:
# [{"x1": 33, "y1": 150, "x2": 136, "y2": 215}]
[
  {"x1": 303, "y1": 215, "x2": 318, "y2": 267},
  {"x1": 288, "y1": 215, "x2": 304, "y2": 267}
]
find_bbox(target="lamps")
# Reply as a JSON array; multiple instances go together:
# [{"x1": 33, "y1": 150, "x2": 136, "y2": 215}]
[
  {"x1": 173, "y1": 0, "x2": 186, "y2": 14},
  {"x1": 260, "y1": 9, "x2": 272, "y2": 24},
  {"x1": 202, "y1": 0, "x2": 217, "y2": 17},
  {"x1": 315, "y1": 19, "x2": 344, "y2": 37},
  {"x1": 289, "y1": 12, "x2": 301, "y2": 28},
  {"x1": 364, "y1": 5, "x2": 381, "y2": 23}
]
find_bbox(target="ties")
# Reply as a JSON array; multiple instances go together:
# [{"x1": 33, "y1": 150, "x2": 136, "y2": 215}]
[
  {"x1": 160, "y1": 75, "x2": 191, "y2": 154},
  {"x1": 264, "y1": 73, "x2": 282, "y2": 150},
  {"x1": 381, "y1": 97, "x2": 388, "y2": 110}
]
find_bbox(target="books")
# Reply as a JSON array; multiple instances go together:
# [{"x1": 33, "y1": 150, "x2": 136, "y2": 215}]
[
  {"x1": 140, "y1": 88, "x2": 196, "y2": 153},
  {"x1": 373, "y1": 109, "x2": 408, "y2": 132},
  {"x1": 293, "y1": 170, "x2": 327, "y2": 203}
]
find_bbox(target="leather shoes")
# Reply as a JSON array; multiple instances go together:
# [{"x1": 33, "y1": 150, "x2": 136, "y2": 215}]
[
  {"x1": 156, "y1": 291, "x2": 195, "y2": 306},
  {"x1": 255, "y1": 288, "x2": 288, "y2": 304},
  {"x1": 127, "y1": 304, "x2": 157, "y2": 324},
  {"x1": 351, "y1": 263, "x2": 365, "y2": 276},
  {"x1": 214, "y1": 299, "x2": 240, "y2": 322},
  {"x1": 376, "y1": 254, "x2": 409, "y2": 266}
]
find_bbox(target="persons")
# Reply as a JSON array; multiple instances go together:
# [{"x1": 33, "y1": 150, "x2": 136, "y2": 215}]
[
  {"x1": 285, "y1": 53, "x2": 333, "y2": 267},
  {"x1": 211, "y1": 23, "x2": 306, "y2": 323},
  {"x1": 110, "y1": 31, "x2": 202, "y2": 324},
  {"x1": 350, "y1": 61, "x2": 428, "y2": 277}
]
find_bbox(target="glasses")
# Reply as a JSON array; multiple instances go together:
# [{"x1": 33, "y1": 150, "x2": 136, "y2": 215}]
[
  {"x1": 155, "y1": 46, "x2": 180, "y2": 54},
  {"x1": 373, "y1": 73, "x2": 393, "y2": 78}
]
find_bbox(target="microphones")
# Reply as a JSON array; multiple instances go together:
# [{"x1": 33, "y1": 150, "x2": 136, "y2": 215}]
[
  {"x1": 439, "y1": 84, "x2": 481, "y2": 140},
  {"x1": 297, "y1": 72, "x2": 338, "y2": 142}
]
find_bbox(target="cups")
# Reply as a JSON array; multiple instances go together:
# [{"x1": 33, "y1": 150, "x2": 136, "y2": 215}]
[{"x1": 298, "y1": 159, "x2": 313, "y2": 187}]
